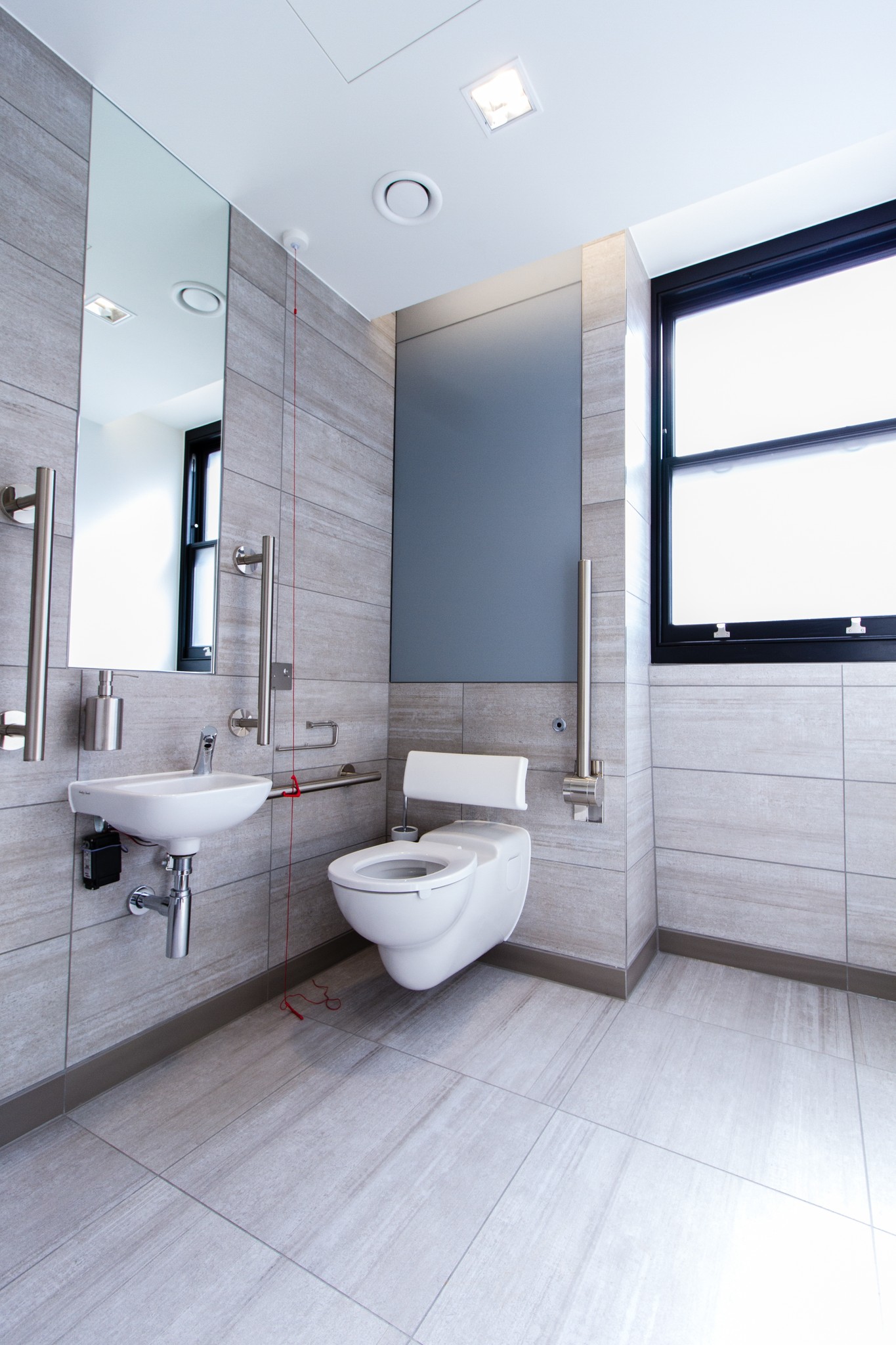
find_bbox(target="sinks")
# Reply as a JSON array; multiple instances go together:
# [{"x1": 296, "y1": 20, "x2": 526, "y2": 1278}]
[{"x1": 69, "y1": 767, "x2": 273, "y2": 841}]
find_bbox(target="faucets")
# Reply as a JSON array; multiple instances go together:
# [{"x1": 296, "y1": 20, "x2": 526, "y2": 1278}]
[{"x1": 192, "y1": 725, "x2": 219, "y2": 775}]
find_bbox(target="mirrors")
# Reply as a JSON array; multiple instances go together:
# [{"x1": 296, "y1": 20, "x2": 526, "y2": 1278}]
[{"x1": 67, "y1": 86, "x2": 231, "y2": 674}]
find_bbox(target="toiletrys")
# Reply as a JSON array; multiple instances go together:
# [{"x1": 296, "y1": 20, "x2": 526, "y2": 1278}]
[{"x1": 83, "y1": 669, "x2": 140, "y2": 753}]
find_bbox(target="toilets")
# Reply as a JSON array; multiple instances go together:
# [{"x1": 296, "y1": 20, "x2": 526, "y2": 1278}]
[{"x1": 328, "y1": 818, "x2": 531, "y2": 991}]
